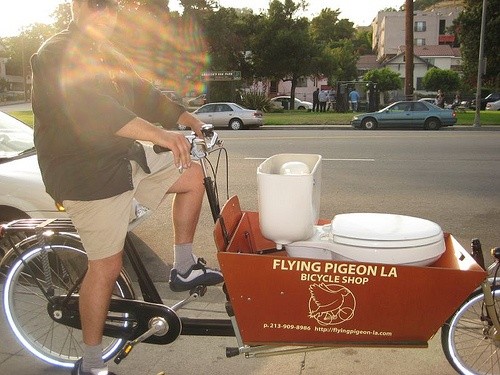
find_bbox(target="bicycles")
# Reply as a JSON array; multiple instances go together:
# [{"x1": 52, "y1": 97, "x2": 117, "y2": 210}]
[{"x1": 1, "y1": 123, "x2": 500, "y2": 375}]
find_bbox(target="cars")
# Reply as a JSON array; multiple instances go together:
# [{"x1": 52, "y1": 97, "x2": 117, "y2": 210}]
[
  {"x1": 271, "y1": 96, "x2": 313, "y2": 110},
  {"x1": 176, "y1": 102, "x2": 263, "y2": 130},
  {"x1": 470, "y1": 93, "x2": 500, "y2": 111},
  {"x1": 485, "y1": 100, "x2": 500, "y2": 110},
  {"x1": 352, "y1": 100, "x2": 457, "y2": 130},
  {"x1": 419, "y1": 97, "x2": 452, "y2": 108},
  {"x1": 188, "y1": 93, "x2": 206, "y2": 106},
  {"x1": 0, "y1": 113, "x2": 70, "y2": 286}
]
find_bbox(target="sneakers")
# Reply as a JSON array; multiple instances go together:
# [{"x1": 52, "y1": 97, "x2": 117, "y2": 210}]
[
  {"x1": 72, "y1": 358, "x2": 118, "y2": 375},
  {"x1": 169, "y1": 257, "x2": 224, "y2": 292}
]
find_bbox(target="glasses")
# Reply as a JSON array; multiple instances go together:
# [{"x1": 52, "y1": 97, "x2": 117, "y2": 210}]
[{"x1": 88, "y1": 0, "x2": 118, "y2": 16}]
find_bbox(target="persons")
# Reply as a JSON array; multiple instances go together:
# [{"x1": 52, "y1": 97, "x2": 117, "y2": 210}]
[
  {"x1": 31, "y1": 1, "x2": 225, "y2": 375},
  {"x1": 318, "y1": 89, "x2": 329, "y2": 112},
  {"x1": 327, "y1": 87, "x2": 337, "y2": 111},
  {"x1": 348, "y1": 88, "x2": 360, "y2": 112},
  {"x1": 452, "y1": 95, "x2": 459, "y2": 105},
  {"x1": 436, "y1": 90, "x2": 444, "y2": 106},
  {"x1": 311, "y1": 88, "x2": 320, "y2": 113}
]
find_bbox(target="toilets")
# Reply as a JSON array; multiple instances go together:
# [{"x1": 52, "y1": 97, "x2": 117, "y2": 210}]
[{"x1": 256, "y1": 154, "x2": 447, "y2": 267}]
